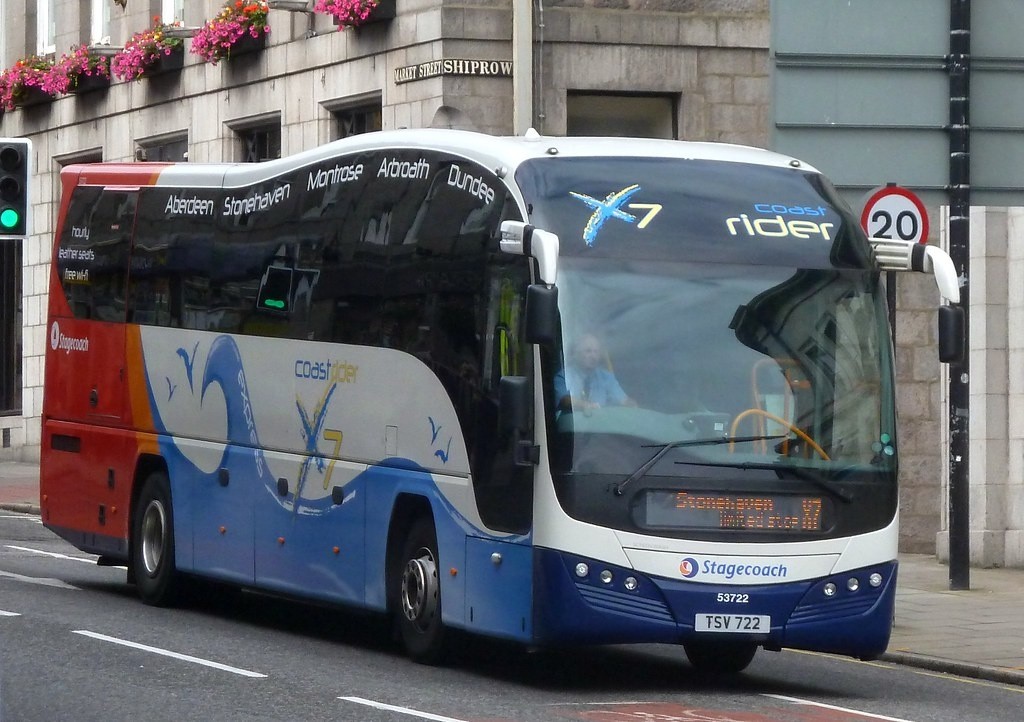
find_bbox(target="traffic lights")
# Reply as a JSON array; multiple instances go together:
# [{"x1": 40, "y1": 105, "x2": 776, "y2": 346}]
[
  {"x1": 0, "y1": 136, "x2": 34, "y2": 239},
  {"x1": 255, "y1": 265, "x2": 292, "y2": 313}
]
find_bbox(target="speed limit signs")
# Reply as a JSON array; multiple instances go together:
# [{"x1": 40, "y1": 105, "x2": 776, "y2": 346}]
[{"x1": 861, "y1": 185, "x2": 930, "y2": 247}]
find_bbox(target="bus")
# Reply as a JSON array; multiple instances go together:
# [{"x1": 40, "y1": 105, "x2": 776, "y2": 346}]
[{"x1": 40, "y1": 123, "x2": 961, "y2": 676}]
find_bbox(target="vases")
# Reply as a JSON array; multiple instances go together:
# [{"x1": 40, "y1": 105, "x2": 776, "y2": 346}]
[
  {"x1": 78, "y1": 78, "x2": 105, "y2": 94},
  {"x1": 143, "y1": 46, "x2": 184, "y2": 78},
  {"x1": 333, "y1": 0, "x2": 396, "y2": 25},
  {"x1": 17, "y1": 88, "x2": 50, "y2": 107},
  {"x1": 222, "y1": 27, "x2": 265, "y2": 57}
]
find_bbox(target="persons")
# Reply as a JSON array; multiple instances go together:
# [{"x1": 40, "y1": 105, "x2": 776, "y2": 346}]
[{"x1": 552, "y1": 331, "x2": 639, "y2": 417}]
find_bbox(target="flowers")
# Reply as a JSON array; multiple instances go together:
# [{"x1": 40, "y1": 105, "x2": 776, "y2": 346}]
[
  {"x1": 40, "y1": 43, "x2": 110, "y2": 97},
  {"x1": 0, "y1": 54, "x2": 54, "y2": 112},
  {"x1": 110, "y1": 15, "x2": 183, "y2": 85},
  {"x1": 313, "y1": 0, "x2": 380, "y2": 33},
  {"x1": 189, "y1": 0, "x2": 270, "y2": 67}
]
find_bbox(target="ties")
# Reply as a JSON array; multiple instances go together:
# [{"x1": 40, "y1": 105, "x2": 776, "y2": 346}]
[{"x1": 583, "y1": 377, "x2": 591, "y2": 400}]
